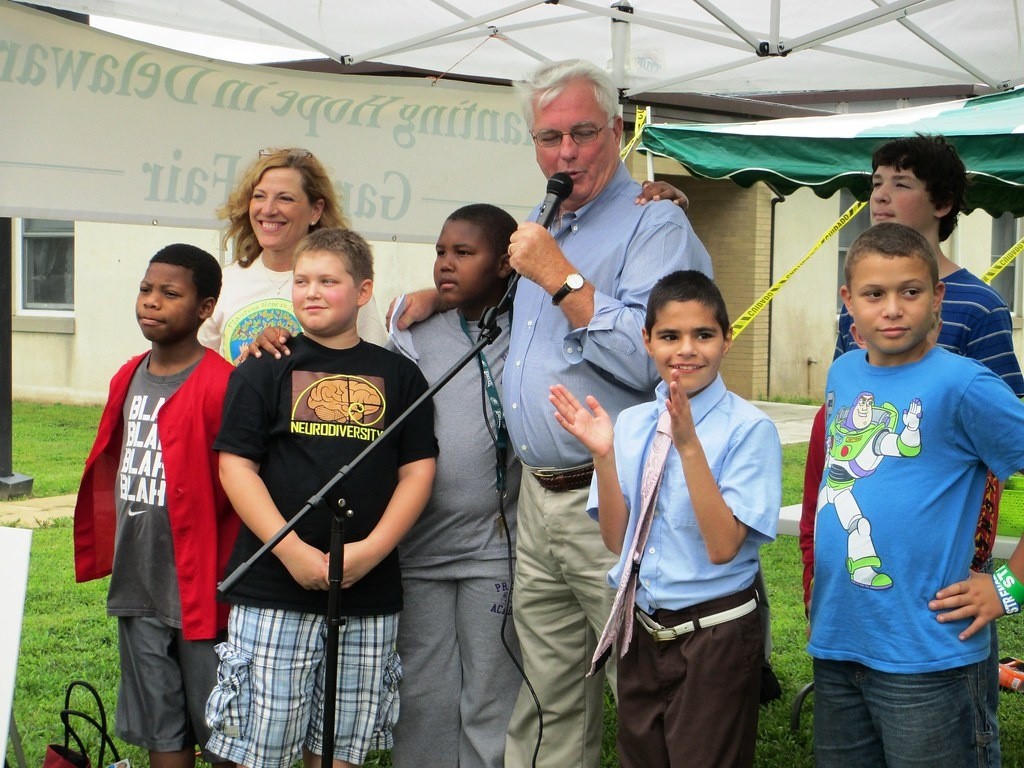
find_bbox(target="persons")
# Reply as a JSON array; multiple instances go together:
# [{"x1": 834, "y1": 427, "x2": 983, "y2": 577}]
[
  {"x1": 72, "y1": 243, "x2": 238, "y2": 768},
  {"x1": 196, "y1": 147, "x2": 388, "y2": 364},
  {"x1": 234, "y1": 178, "x2": 689, "y2": 768},
  {"x1": 547, "y1": 270, "x2": 784, "y2": 768},
  {"x1": 807, "y1": 223, "x2": 1024, "y2": 768},
  {"x1": 206, "y1": 229, "x2": 440, "y2": 768},
  {"x1": 799, "y1": 301, "x2": 1004, "y2": 642},
  {"x1": 385, "y1": 58, "x2": 714, "y2": 767},
  {"x1": 832, "y1": 130, "x2": 1022, "y2": 394}
]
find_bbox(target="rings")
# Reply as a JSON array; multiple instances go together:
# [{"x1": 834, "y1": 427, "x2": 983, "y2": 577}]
[{"x1": 508, "y1": 243, "x2": 514, "y2": 257}]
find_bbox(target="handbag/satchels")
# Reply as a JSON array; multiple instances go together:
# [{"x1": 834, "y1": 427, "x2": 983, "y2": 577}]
[{"x1": 41, "y1": 680, "x2": 120, "y2": 768}]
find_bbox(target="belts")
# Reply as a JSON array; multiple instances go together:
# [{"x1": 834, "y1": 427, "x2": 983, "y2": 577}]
[
  {"x1": 531, "y1": 464, "x2": 594, "y2": 491},
  {"x1": 633, "y1": 588, "x2": 760, "y2": 642}
]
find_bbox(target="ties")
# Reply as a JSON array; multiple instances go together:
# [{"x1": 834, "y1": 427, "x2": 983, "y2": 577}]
[{"x1": 585, "y1": 409, "x2": 673, "y2": 682}]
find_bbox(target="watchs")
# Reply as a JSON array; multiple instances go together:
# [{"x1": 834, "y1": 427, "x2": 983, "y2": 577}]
[{"x1": 552, "y1": 272, "x2": 586, "y2": 306}]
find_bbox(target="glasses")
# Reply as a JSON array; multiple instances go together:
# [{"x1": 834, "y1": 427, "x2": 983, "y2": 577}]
[
  {"x1": 530, "y1": 119, "x2": 612, "y2": 147},
  {"x1": 258, "y1": 148, "x2": 313, "y2": 160}
]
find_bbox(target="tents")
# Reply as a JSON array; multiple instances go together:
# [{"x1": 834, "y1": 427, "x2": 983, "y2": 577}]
[{"x1": 639, "y1": 83, "x2": 1023, "y2": 224}]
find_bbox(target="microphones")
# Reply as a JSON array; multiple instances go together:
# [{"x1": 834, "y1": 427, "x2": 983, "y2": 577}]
[{"x1": 535, "y1": 173, "x2": 573, "y2": 229}]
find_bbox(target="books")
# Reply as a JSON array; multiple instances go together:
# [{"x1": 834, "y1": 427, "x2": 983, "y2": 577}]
[{"x1": 389, "y1": 295, "x2": 419, "y2": 363}]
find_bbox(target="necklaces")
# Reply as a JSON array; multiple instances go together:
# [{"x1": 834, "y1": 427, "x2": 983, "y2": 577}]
[{"x1": 260, "y1": 253, "x2": 293, "y2": 294}]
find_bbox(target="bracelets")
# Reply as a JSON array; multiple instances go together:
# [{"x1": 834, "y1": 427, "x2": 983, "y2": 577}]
[{"x1": 992, "y1": 563, "x2": 1024, "y2": 616}]
